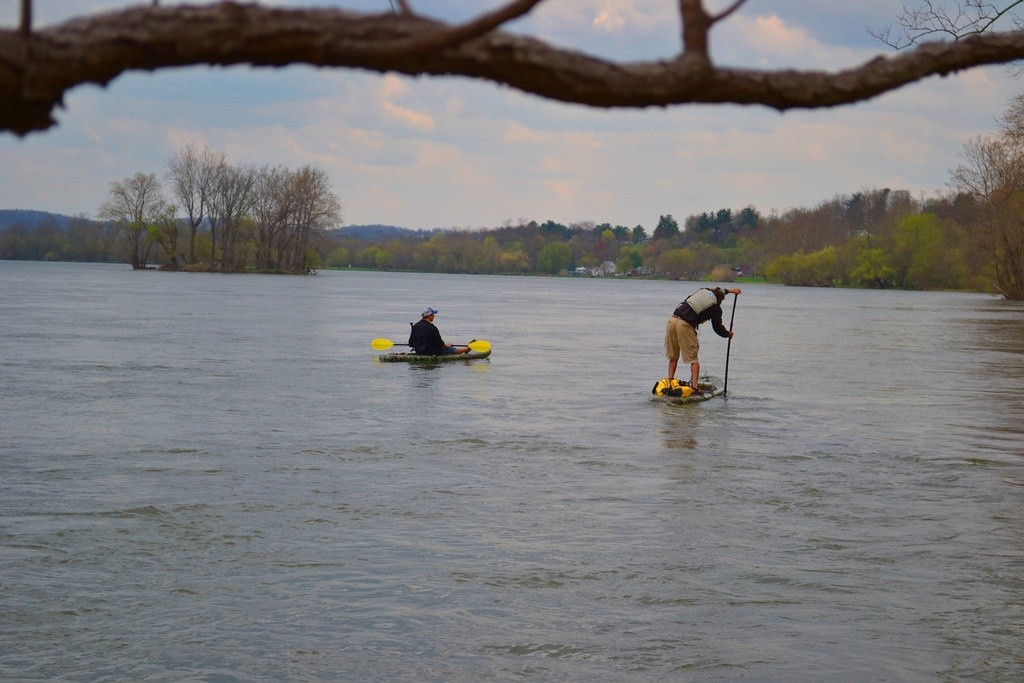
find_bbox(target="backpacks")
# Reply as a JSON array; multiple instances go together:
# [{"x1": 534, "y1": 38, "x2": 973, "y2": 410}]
[{"x1": 675, "y1": 288, "x2": 718, "y2": 314}]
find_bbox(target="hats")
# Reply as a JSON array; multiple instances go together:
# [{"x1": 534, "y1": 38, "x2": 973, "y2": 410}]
[{"x1": 421, "y1": 308, "x2": 438, "y2": 316}]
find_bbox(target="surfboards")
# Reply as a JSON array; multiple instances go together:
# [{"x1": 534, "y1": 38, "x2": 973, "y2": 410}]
[
  {"x1": 650, "y1": 375, "x2": 725, "y2": 402},
  {"x1": 379, "y1": 350, "x2": 491, "y2": 362}
]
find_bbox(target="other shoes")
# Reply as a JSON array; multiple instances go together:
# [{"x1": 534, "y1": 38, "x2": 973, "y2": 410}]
[{"x1": 465, "y1": 340, "x2": 478, "y2": 353}]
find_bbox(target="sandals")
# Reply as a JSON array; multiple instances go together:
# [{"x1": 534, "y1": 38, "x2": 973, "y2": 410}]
[{"x1": 691, "y1": 386, "x2": 704, "y2": 394}]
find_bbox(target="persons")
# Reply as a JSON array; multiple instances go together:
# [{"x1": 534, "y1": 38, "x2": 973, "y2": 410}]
[
  {"x1": 408, "y1": 307, "x2": 476, "y2": 357},
  {"x1": 664, "y1": 287, "x2": 741, "y2": 396}
]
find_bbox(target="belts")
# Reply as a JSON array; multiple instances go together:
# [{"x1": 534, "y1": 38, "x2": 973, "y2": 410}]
[{"x1": 671, "y1": 314, "x2": 684, "y2": 320}]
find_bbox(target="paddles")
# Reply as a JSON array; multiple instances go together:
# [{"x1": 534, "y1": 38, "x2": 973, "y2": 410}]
[
  {"x1": 371, "y1": 338, "x2": 491, "y2": 353},
  {"x1": 723, "y1": 293, "x2": 738, "y2": 396}
]
